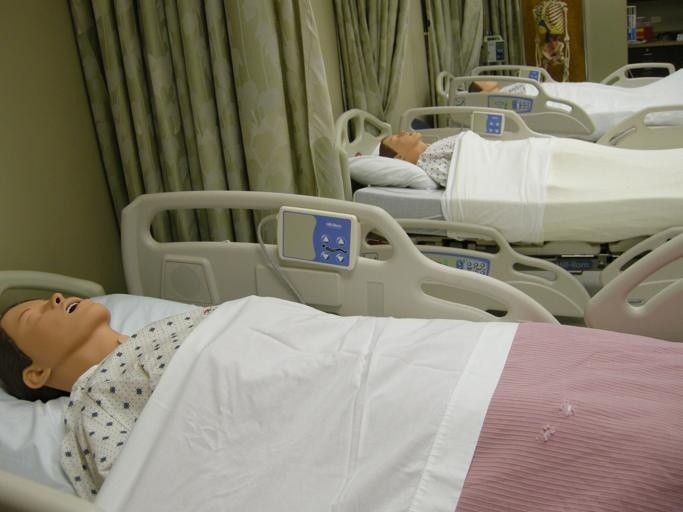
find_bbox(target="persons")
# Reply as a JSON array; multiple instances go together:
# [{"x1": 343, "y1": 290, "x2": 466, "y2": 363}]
[
  {"x1": 0, "y1": 290, "x2": 227, "y2": 501},
  {"x1": 376, "y1": 129, "x2": 466, "y2": 189},
  {"x1": 466, "y1": 80, "x2": 528, "y2": 97}
]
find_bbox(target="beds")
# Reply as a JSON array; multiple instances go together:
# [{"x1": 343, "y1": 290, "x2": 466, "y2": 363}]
[
  {"x1": 434, "y1": 61, "x2": 683, "y2": 142},
  {"x1": 333, "y1": 109, "x2": 683, "y2": 296},
  {"x1": 0, "y1": 189, "x2": 683, "y2": 512}
]
